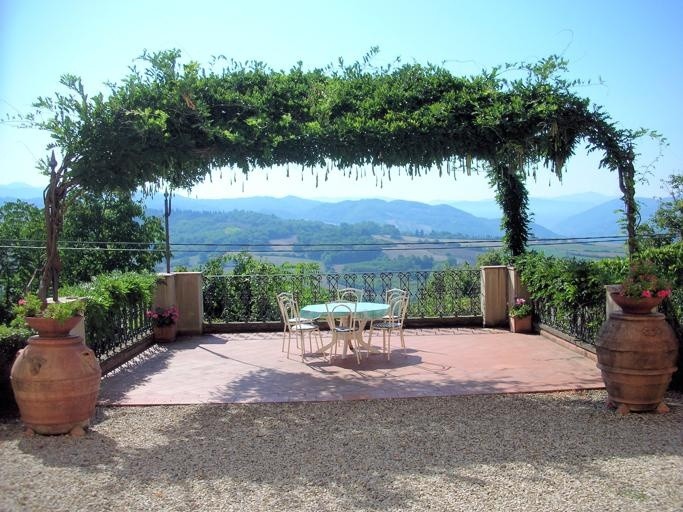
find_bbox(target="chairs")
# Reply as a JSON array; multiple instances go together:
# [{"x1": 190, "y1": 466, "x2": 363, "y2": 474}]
[{"x1": 277, "y1": 287, "x2": 409, "y2": 364}]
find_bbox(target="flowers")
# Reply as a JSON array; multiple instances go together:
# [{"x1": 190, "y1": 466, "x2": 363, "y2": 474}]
[
  {"x1": 17, "y1": 294, "x2": 86, "y2": 325},
  {"x1": 509, "y1": 298, "x2": 531, "y2": 319},
  {"x1": 147, "y1": 305, "x2": 179, "y2": 326},
  {"x1": 618, "y1": 260, "x2": 672, "y2": 300}
]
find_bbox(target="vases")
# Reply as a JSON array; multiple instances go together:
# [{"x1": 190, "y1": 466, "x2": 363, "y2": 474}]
[
  {"x1": 611, "y1": 292, "x2": 663, "y2": 314},
  {"x1": 153, "y1": 325, "x2": 175, "y2": 342},
  {"x1": 509, "y1": 314, "x2": 531, "y2": 333},
  {"x1": 23, "y1": 315, "x2": 82, "y2": 336}
]
